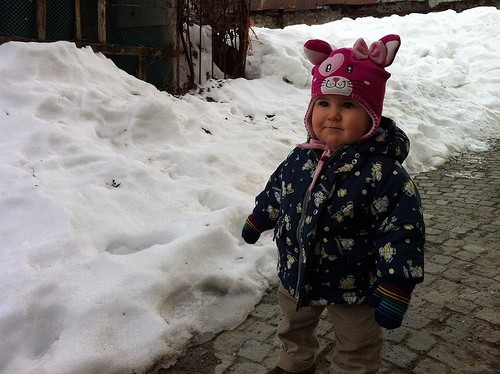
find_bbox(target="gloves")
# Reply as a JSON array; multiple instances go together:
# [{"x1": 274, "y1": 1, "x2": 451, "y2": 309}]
[
  {"x1": 364, "y1": 272, "x2": 413, "y2": 330},
  {"x1": 240, "y1": 195, "x2": 283, "y2": 246}
]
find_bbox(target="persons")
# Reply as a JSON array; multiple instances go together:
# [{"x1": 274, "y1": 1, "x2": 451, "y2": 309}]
[{"x1": 241, "y1": 34, "x2": 425, "y2": 374}]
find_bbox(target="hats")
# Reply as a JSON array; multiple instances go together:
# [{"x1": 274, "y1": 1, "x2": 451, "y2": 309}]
[{"x1": 302, "y1": 33, "x2": 401, "y2": 150}]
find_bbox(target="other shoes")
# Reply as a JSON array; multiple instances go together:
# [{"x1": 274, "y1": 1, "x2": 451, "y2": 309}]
[{"x1": 264, "y1": 361, "x2": 317, "y2": 374}]
